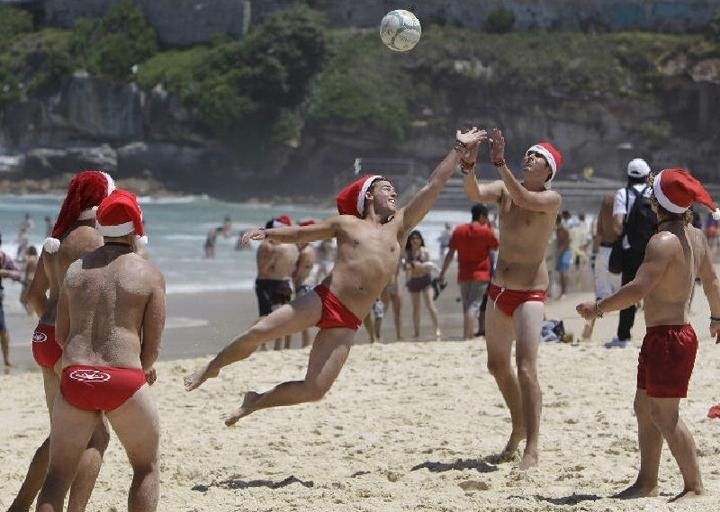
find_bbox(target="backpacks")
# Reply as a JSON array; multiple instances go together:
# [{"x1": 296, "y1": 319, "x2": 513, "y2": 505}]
[{"x1": 627, "y1": 186, "x2": 657, "y2": 256}]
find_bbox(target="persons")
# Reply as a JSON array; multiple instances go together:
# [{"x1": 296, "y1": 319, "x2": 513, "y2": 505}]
[
  {"x1": 463, "y1": 125, "x2": 564, "y2": 470},
  {"x1": 366, "y1": 208, "x2": 498, "y2": 344},
  {"x1": 0, "y1": 171, "x2": 165, "y2": 512},
  {"x1": 255, "y1": 215, "x2": 335, "y2": 350},
  {"x1": 577, "y1": 169, "x2": 720, "y2": 504},
  {"x1": 608, "y1": 157, "x2": 665, "y2": 347},
  {"x1": 545, "y1": 202, "x2": 638, "y2": 339},
  {"x1": 204, "y1": 217, "x2": 251, "y2": 258},
  {"x1": 183, "y1": 126, "x2": 486, "y2": 427},
  {"x1": 693, "y1": 207, "x2": 720, "y2": 247}
]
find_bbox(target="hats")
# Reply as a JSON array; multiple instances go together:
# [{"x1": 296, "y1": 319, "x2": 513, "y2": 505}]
[
  {"x1": 653, "y1": 169, "x2": 720, "y2": 221},
  {"x1": 43, "y1": 171, "x2": 147, "y2": 253},
  {"x1": 627, "y1": 158, "x2": 651, "y2": 178},
  {"x1": 525, "y1": 143, "x2": 561, "y2": 189},
  {"x1": 336, "y1": 175, "x2": 382, "y2": 216},
  {"x1": 273, "y1": 216, "x2": 290, "y2": 227}
]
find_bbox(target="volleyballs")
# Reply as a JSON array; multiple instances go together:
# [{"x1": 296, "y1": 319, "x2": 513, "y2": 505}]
[{"x1": 381, "y1": 9, "x2": 421, "y2": 53}]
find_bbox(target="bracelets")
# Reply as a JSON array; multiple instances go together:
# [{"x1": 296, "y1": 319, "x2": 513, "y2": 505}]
[
  {"x1": 453, "y1": 140, "x2": 465, "y2": 154},
  {"x1": 494, "y1": 158, "x2": 508, "y2": 167},
  {"x1": 461, "y1": 162, "x2": 475, "y2": 168},
  {"x1": 711, "y1": 316, "x2": 720, "y2": 321}
]
